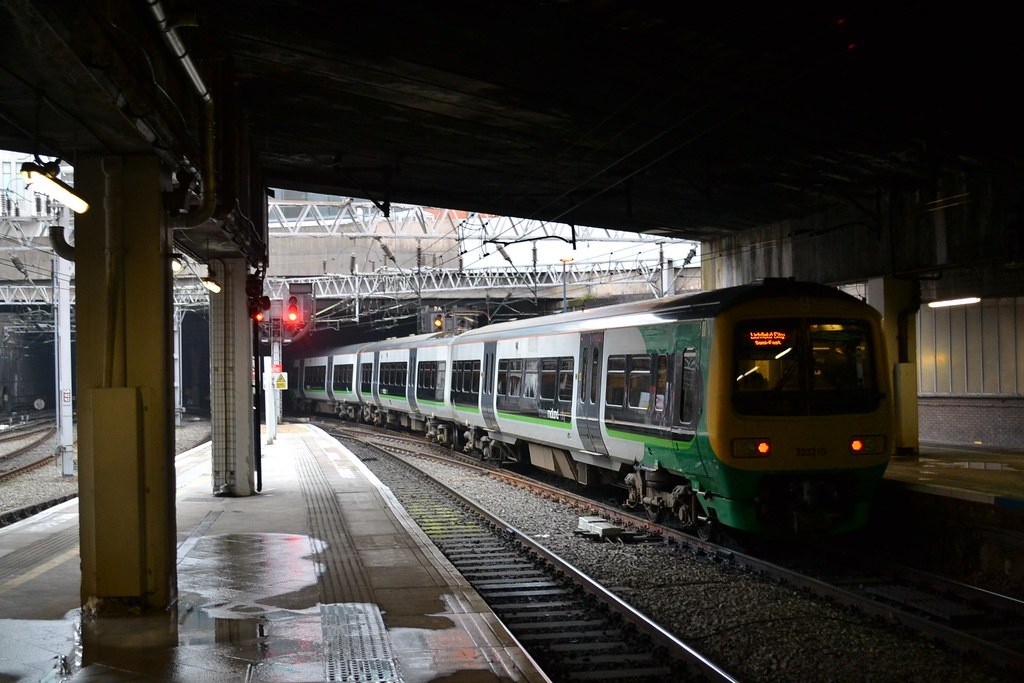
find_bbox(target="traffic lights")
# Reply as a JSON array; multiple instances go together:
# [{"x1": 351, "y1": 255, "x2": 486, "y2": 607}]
[
  {"x1": 429, "y1": 312, "x2": 446, "y2": 333},
  {"x1": 283, "y1": 293, "x2": 304, "y2": 323}
]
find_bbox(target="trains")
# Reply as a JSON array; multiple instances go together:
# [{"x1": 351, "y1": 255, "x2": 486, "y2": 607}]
[{"x1": 283, "y1": 275, "x2": 900, "y2": 540}]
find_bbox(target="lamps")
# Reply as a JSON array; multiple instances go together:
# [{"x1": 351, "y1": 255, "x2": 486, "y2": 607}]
[
  {"x1": 21, "y1": 161, "x2": 89, "y2": 214},
  {"x1": 172, "y1": 257, "x2": 182, "y2": 271},
  {"x1": 202, "y1": 279, "x2": 222, "y2": 293}
]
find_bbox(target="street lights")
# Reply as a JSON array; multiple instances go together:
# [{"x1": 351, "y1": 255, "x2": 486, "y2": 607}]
[{"x1": 246, "y1": 274, "x2": 271, "y2": 493}]
